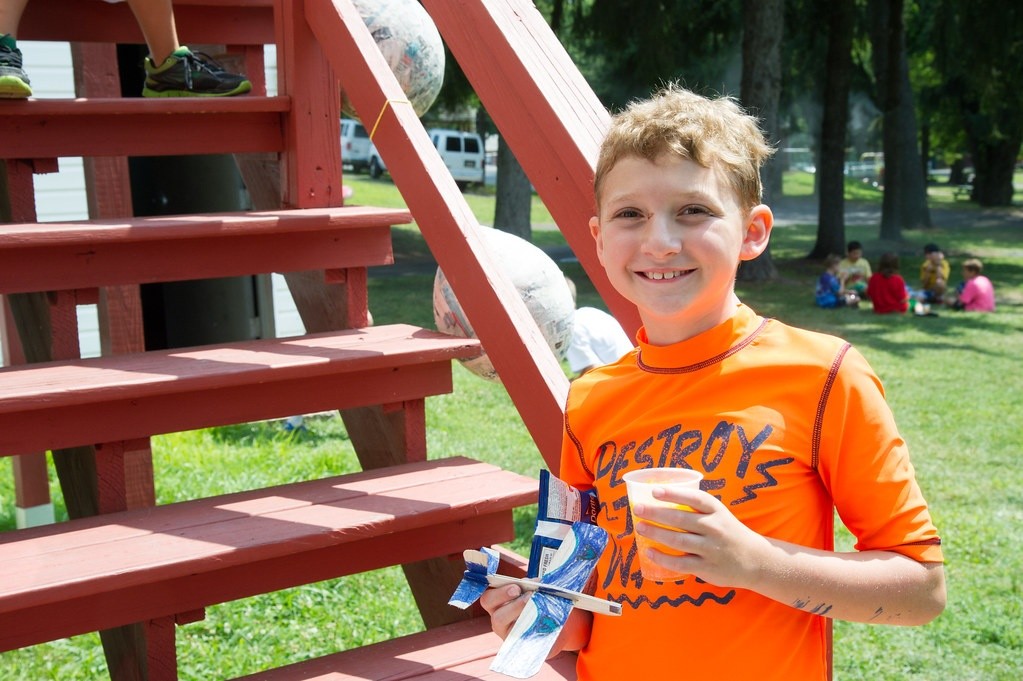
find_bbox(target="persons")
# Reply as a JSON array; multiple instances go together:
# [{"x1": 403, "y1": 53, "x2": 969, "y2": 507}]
[
  {"x1": 920, "y1": 243, "x2": 949, "y2": 302},
  {"x1": 0, "y1": 0, "x2": 253, "y2": 98},
  {"x1": 836, "y1": 241, "x2": 872, "y2": 295},
  {"x1": 479, "y1": 89, "x2": 946, "y2": 681},
  {"x1": 816, "y1": 257, "x2": 860, "y2": 308},
  {"x1": 947, "y1": 258, "x2": 995, "y2": 312},
  {"x1": 866, "y1": 252, "x2": 914, "y2": 312}
]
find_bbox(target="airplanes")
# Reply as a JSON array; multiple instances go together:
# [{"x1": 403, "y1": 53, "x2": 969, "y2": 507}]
[{"x1": 446, "y1": 519, "x2": 623, "y2": 680}]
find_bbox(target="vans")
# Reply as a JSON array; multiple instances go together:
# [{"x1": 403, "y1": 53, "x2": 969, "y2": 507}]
[
  {"x1": 341, "y1": 121, "x2": 371, "y2": 173},
  {"x1": 368, "y1": 130, "x2": 484, "y2": 193}
]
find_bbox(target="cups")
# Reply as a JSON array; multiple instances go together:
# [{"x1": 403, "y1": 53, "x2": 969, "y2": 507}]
[{"x1": 622, "y1": 467, "x2": 704, "y2": 582}]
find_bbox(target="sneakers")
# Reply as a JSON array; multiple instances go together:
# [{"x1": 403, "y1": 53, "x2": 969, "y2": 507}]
[
  {"x1": 142, "y1": 46, "x2": 252, "y2": 97},
  {"x1": 0, "y1": 33, "x2": 32, "y2": 98}
]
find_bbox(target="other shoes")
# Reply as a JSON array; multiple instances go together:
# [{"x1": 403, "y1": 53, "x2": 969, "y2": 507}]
[{"x1": 915, "y1": 310, "x2": 937, "y2": 317}]
